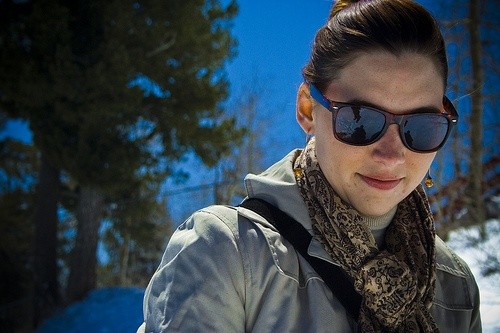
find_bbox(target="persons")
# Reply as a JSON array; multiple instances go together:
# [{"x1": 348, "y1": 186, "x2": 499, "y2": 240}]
[{"x1": 128, "y1": 0, "x2": 484, "y2": 333}]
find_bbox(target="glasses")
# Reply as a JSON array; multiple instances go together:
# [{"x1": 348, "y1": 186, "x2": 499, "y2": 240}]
[{"x1": 306, "y1": 82, "x2": 460, "y2": 152}]
